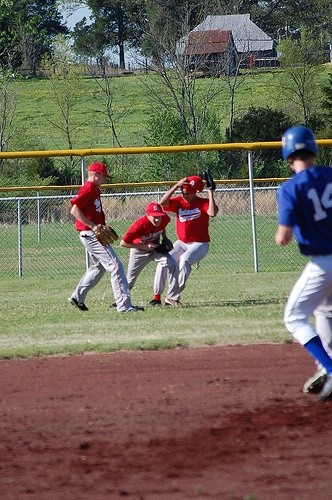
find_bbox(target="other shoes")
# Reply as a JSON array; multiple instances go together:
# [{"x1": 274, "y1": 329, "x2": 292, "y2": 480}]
[
  {"x1": 303, "y1": 369, "x2": 332, "y2": 402},
  {"x1": 149, "y1": 299, "x2": 162, "y2": 307}
]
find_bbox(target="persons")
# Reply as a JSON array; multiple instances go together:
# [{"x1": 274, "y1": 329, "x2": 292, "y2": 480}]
[
  {"x1": 275, "y1": 128, "x2": 332, "y2": 400},
  {"x1": 68, "y1": 162, "x2": 144, "y2": 313},
  {"x1": 150, "y1": 170, "x2": 218, "y2": 306},
  {"x1": 112, "y1": 203, "x2": 184, "y2": 307}
]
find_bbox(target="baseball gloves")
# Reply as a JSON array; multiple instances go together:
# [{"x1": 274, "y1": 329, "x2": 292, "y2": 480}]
[
  {"x1": 202, "y1": 169, "x2": 217, "y2": 191},
  {"x1": 154, "y1": 236, "x2": 174, "y2": 255},
  {"x1": 95, "y1": 224, "x2": 119, "y2": 247}
]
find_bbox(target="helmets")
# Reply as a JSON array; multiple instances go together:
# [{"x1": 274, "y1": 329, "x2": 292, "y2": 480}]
[{"x1": 281, "y1": 126, "x2": 318, "y2": 160}]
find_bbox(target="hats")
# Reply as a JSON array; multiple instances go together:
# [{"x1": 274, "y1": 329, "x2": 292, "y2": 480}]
[
  {"x1": 186, "y1": 175, "x2": 205, "y2": 192},
  {"x1": 88, "y1": 163, "x2": 112, "y2": 178},
  {"x1": 145, "y1": 202, "x2": 167, "y2": 216}
]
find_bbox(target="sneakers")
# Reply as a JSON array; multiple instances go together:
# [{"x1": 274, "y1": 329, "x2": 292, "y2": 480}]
[
  {"x1": 121, "y1": 305, "x2": 145, "y2": 313},
  {"x1": 108, "y1": 303, "x2": 117, "y2": 309},
  {"x1": 165, "y1": 298, "x2": 183, "y2": 307},
  {"x1": 68, "y1": 296, "x2": 88, "y2": 311}
]
